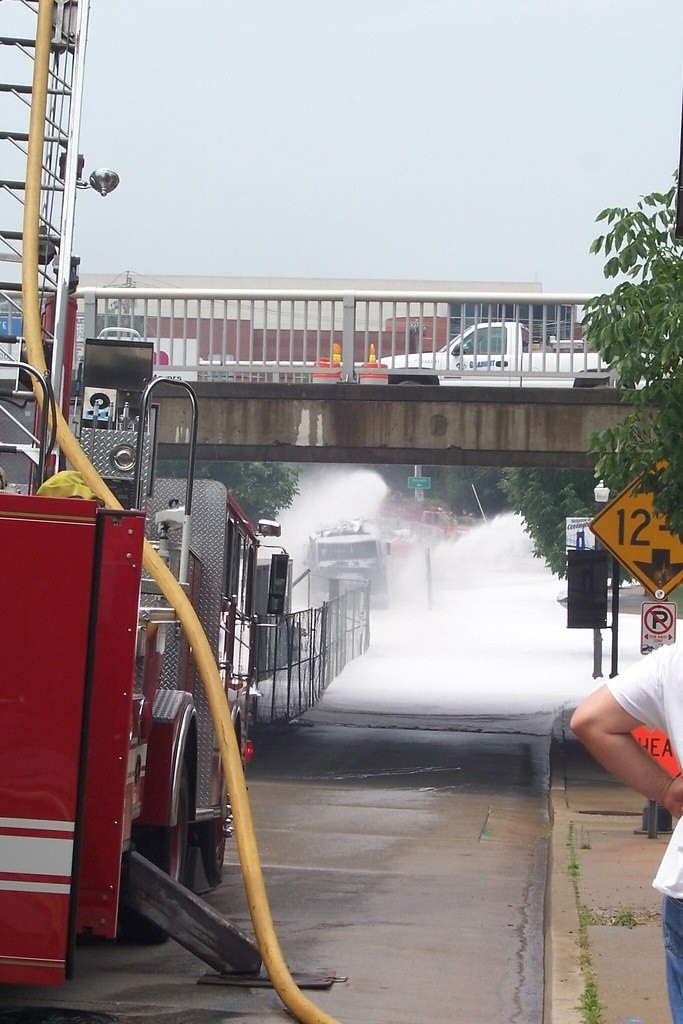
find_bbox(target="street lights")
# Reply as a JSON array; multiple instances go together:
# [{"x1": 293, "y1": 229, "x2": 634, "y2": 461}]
[{"x1": 591, "y1": 479, "x2": 610, "y2": 679}]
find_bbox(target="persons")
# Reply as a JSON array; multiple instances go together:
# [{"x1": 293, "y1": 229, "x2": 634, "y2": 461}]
[{"x1": 569, "y1": 638, "x2": 683, "y2": 1024}]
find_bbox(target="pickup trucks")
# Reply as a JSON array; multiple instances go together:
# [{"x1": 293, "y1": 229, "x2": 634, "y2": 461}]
[{"x1": 376, "y1": 320, "x2": 619, "y2": 389}]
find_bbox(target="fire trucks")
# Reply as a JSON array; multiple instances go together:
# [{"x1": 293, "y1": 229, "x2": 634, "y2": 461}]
[
  {"x1": 304, "y1": 500, "x2": 475, "y2": 613},
  {"x1": 0, "y1": 0, "x2": 285, "y2": 989}
]
